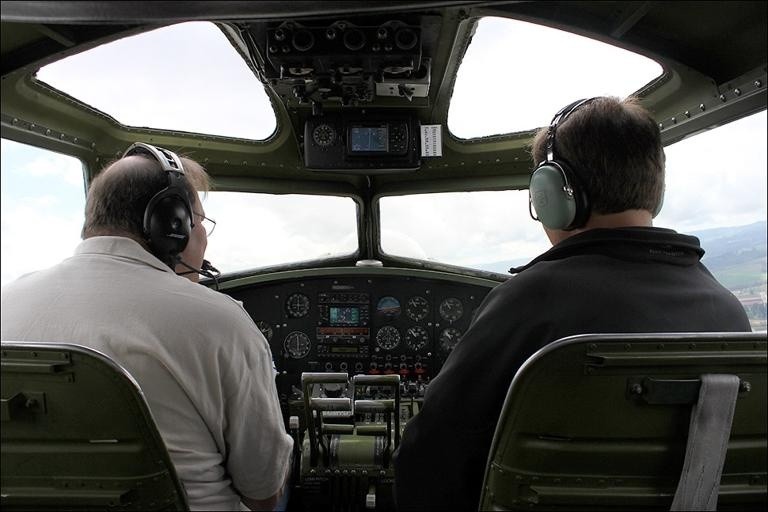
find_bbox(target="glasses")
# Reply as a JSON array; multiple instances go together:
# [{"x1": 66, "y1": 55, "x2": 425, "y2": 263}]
[{"x1": 193, "y1": 211, "x2": 216, "y2": 237}]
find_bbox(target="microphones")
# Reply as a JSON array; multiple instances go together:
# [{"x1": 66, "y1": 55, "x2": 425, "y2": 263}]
[{"x1": 176, "y1": 253, "x2": 222, "y2": 279}]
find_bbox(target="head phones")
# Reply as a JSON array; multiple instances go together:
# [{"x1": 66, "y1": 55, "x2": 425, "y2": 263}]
[
  {"x1": 118, "y1": 141, "x2": 195, "y2": 257},
  {"x1": 529, "y1": 97, "x2": 602, "y2": 233}
]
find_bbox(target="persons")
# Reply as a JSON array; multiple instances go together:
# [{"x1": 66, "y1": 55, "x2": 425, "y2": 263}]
[
  {"x1": 392, "y1": 99, "x2": 754, "y2": 511},
  {"x1": 1, "y1": 151, "x2": 294, "y2": 511}
]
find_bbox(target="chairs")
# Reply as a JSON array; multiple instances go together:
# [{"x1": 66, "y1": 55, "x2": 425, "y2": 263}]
[
  {"x1": 476, "y1": 330, "x2": 768, "y2": 511},
  {"x1": 1, "y1": 340, "x2": 191, "y2": 511}
]
